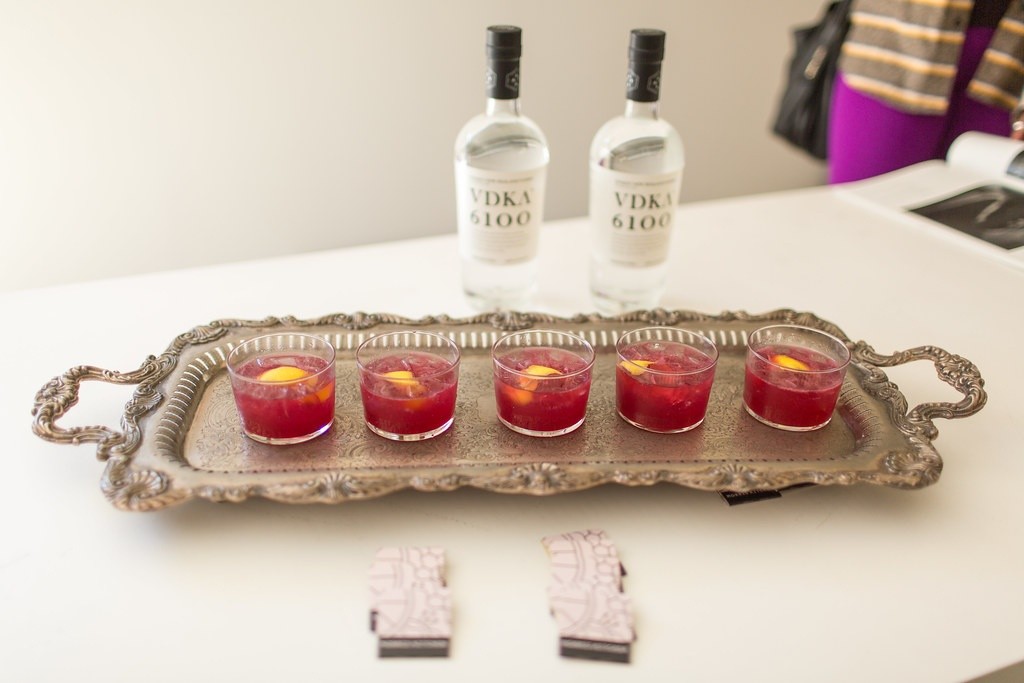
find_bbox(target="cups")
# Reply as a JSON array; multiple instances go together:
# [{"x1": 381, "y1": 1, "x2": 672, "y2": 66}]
[
  {"x1": 356, "y1": 332, "x2": 462, "y2": 442},
  {"x1": 494, "y1": 330, "x2": 596, "y2": 437},
  {"x1": 226, "y1": 331, "x2": 338, "y2": 447},
  {"x1": 746, "y1": 324, "x2": 852, "y2": 433},
  {"x1": 616, "y1": 328, "x2": 717, "y2": 433}
]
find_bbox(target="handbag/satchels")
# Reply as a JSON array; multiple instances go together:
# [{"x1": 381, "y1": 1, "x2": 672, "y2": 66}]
[{"x1": 767, "y1": 1, "x2": 855, "y2": 159}]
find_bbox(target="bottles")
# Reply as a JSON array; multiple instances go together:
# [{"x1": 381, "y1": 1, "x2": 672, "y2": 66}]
[
  {"x1": 586, "y1": 29, "x2": 686, "y2": 312},
  {"x1": 455, "y1": 25, "x2": 548, "y2": 299}
]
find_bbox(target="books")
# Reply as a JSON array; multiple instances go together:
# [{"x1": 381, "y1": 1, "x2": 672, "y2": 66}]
[{"x1": 833, "y1": 130, "x2": 1024, "y2": 265}]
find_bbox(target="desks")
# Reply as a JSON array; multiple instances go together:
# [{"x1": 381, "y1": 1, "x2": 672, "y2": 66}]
[{"x1": 0, "y1": 180, "x2": 1024, "y2": 682}]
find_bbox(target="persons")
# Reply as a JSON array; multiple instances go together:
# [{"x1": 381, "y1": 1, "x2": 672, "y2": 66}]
[{"x1": 828, "y1": 0, "x2": 1024, "y2": 185}]
